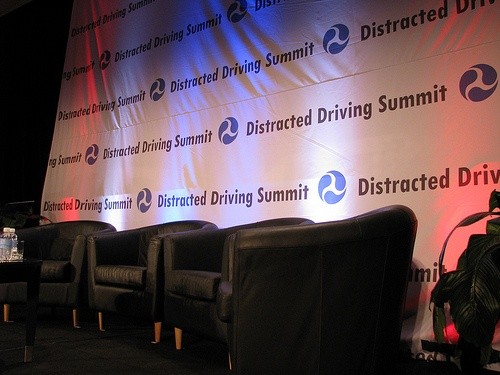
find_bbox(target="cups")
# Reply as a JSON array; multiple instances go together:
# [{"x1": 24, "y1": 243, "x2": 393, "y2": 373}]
[{"x1": 12, "y1": 240, "x2": 24, "y2": 260}]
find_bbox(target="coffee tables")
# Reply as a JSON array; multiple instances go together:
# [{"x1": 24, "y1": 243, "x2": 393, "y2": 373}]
[{"x1": 0, "y1": 259, "x2": 43, "y2": 362}]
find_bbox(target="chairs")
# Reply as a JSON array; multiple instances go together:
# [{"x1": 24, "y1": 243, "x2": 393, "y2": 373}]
[
  {"x1": 217, "y1": 204, "x2": 418, "y2": 375},
  {"x1": 0, "y1": 220, "x2": 116, "y2": 327},
  {"x1": 164, "y1": 216, "x2": 315, "y2": 349},
  {"x1": 87, "y1": 220, "x2": 218, "y2": 342}
]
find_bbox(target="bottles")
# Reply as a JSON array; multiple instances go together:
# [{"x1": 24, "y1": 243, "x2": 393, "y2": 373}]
[
  {"x1": 0, "y1": 228, "x2": 12, "y2": 261},
  {"x1": 10, "y1": 228, "x2": 17, "y2": 258}
]
